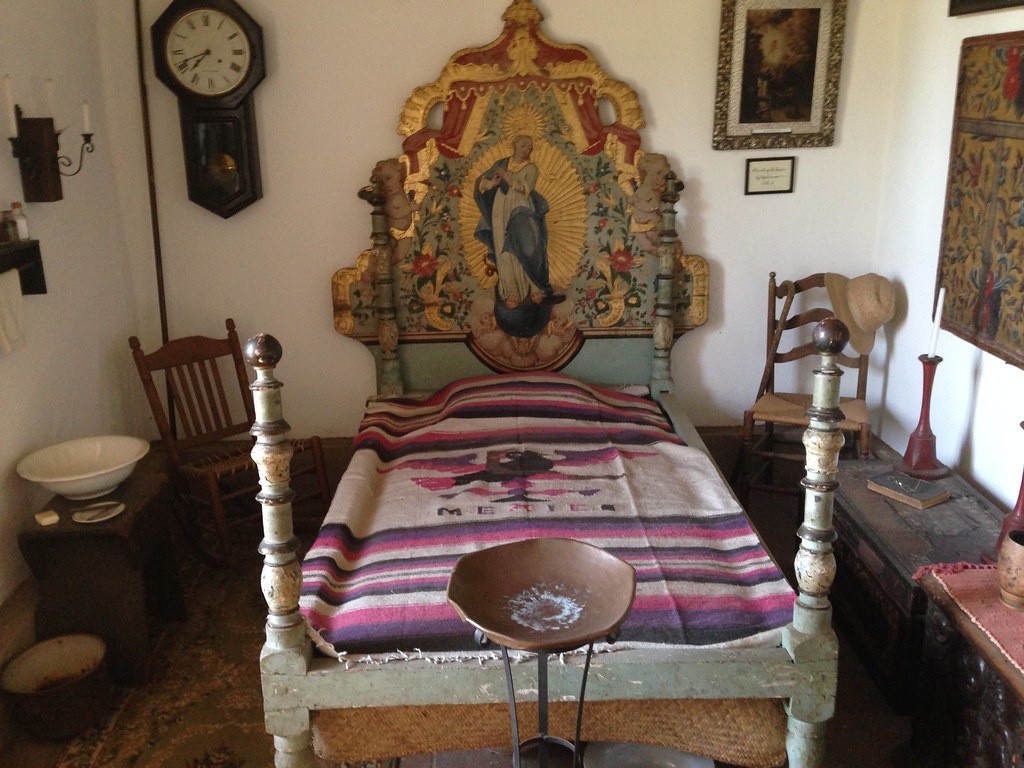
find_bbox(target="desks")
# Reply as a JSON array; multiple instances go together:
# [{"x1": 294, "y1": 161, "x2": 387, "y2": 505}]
[
  {"x1": 17, "y1": 463, "x2": 192, "y2": 685},
  {"x1": 830, "y1": 457, "x2": 1007, "y2": 713},
  {"x1": 911, "y1": 564, "x2": 1024, "y2": 768}
]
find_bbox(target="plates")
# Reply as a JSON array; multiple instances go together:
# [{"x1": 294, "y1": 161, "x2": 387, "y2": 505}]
[{"x1": 71, "y1": 501, "x2": 126, "y2": 523}]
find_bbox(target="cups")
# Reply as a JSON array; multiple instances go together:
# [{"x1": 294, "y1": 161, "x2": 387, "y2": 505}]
[{"x1": 997, "y1": 529, "x2": 1024, "y2": 610}]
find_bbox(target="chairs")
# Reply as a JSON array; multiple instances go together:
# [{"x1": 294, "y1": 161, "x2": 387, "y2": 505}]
[
  {"x1": 739, "y1": 269, "x2": 868, "y2": 509},
  {"x1": 127, "y1": 318, "x2": 333, "y2": 563}
]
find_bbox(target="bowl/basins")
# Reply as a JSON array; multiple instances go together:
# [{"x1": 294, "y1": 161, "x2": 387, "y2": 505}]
[
  {"x1": 0, "y1": 634, "x2": 123, "y2": 742},
  {"x1": 15, "y1": 435, "x2": 150, "y2": 499},
  {"x1": 446, "y1": 537, "x2": 637, "y2": 650}
]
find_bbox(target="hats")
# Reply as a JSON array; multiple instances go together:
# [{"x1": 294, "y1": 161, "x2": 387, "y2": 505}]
[{"x1": 824, "y1": 271, "x2": 896, "y2": 355}]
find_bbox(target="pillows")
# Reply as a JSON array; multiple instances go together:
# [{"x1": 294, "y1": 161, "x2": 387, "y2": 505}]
[{"x1": 439, "y1": 370, "x2": 586, "y2": 431}]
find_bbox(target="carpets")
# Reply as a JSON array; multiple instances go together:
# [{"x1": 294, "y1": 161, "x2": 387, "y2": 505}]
[{"x1": 56, "y1": 543, "x2": 401, "y2": 768}]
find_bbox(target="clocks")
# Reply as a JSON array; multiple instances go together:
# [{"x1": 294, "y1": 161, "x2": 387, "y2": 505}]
[{"x1": 151, "y1": 0, "x2": 269, "y2": 219}]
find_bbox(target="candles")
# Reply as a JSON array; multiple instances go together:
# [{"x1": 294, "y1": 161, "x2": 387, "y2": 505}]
[
  {"x1": 48, "y1": 76, "x2": 61, "y2": 132},
  {"x1": 81, "y1": 99, "x2": 91, "y2": 133},
  {"x1": 928, "y1": 285, "x2": 947, "y2": 356},
  {"x1": 3, "y1": 74, "x2": 19, "y2": 137}
]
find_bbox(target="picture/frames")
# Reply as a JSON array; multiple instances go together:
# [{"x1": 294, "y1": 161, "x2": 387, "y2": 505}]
[
  {"x1": 948, "y1": 0, "x2": 1024, "y2": 17},
  {"x1": 744, "y1": 156, "x2": 796, "y2": 195},
  {"x1": 710, "y1": 0, "x2": 849, "y2": 155},
  {"x1": 931, "y1": 28, "x2": 1024, "y2": 374}
]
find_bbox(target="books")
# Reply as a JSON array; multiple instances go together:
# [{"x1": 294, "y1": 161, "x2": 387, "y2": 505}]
[{"x1": 867, "y1": 469, "x2": 951, "y2": 508}]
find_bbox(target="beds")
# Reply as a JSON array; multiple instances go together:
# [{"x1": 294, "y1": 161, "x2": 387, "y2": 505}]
[{"x1": 245, "y1": 0, "x2": 851, "y2": 768}]
